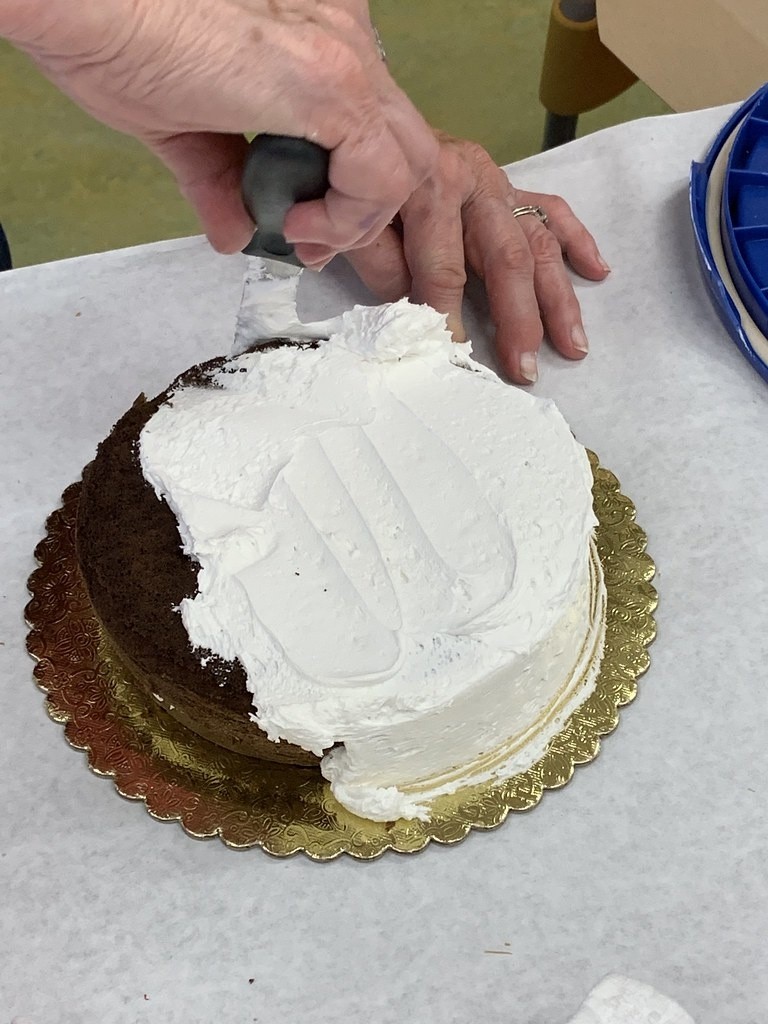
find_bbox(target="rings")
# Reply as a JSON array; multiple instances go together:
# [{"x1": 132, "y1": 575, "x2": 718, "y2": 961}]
[
  {"x1": 372, "y1": 25, "x2": 389, "y2": 64},
  {"x1": 511, "y1": 204, "x2": 549, "y2": 225}
]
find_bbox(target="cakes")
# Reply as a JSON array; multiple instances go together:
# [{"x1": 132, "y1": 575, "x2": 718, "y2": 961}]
[{"x1": 69, "y1": 299, "x2": 607, "y2": 822}]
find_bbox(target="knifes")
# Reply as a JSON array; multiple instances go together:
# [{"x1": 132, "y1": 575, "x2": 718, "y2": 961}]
[{"x1": 225, "y1": 133, "x2": 329, "y2": 357}]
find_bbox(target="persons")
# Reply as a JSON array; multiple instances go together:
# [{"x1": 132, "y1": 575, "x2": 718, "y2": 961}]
[{"x1": 0, "y1": 1, "x2": 610, "y2": 387}]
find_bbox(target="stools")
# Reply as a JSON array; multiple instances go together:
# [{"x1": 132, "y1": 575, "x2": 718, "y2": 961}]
[{"x1": 539, "y1": 0, "x2": 767, "y2": 162}]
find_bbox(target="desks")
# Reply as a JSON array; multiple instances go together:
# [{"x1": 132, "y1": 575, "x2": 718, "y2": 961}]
[{"x1": 1, "y1": 102, "x2": 767, "y2": 1024}]
